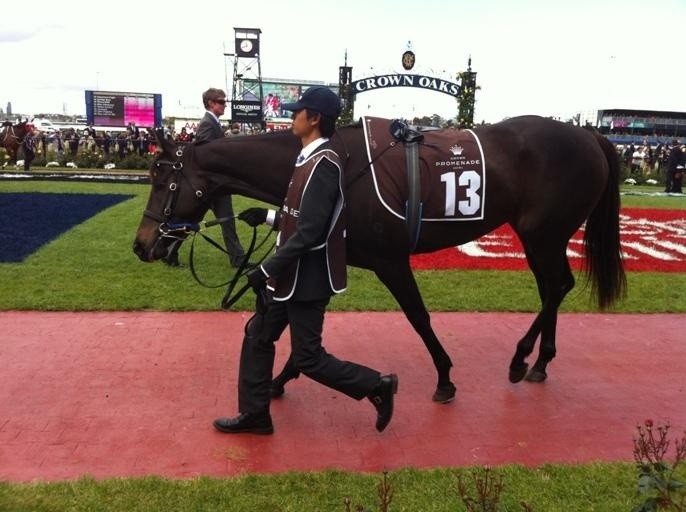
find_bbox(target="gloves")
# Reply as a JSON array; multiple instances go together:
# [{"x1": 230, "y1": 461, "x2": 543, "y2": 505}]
[
  {"x1": 236, "y1": 207, "x2": 268, "y2": 228},
  {"x1": 247, "y1": 268, "x2": 266, "y2": 291}
]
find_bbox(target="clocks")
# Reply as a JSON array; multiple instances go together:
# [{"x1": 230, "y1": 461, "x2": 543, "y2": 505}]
[{"x1": 240, "y1": 40, "x2": 253, "y2": 52}]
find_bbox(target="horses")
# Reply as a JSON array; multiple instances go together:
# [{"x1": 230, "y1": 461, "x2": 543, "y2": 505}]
[
  {"x1": 0, "y1": 117, "x2": 35, "y2": 170},
  {"x1": 132, "y1": 115, "x2": 628, "y2": 404}
]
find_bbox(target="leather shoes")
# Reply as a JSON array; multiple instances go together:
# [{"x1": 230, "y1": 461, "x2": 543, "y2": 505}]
[
  {"x1": 232, "y1": 261, "x2": 258, "y2": 269},
  {"x1": 161, "y1": 256, "x2": 182, "y2": 267},
  {"x1": 368, "y1": 373, "x2": 399, "y2": 432},
  {"x1": 212, "y1": 412, "x2": 274, "y2": 434}
]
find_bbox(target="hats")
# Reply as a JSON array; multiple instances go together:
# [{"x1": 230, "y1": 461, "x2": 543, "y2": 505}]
[
  {"x1": 672, "y1": 140, "x2": 679, "y2": 145},
  {"x1": 281, "y1": 85, "x2": 341, "y2": 118}
]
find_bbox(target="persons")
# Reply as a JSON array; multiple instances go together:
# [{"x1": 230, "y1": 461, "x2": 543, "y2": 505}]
[
  {"x1": 612, "y1": 137, "x2": 686, "y2": 193},
  {"x1": 161, "y1": 88, "x2": 260, "y2": 269},
  {"x1": 0, "y1": 114, "x2": 244, "y2": 174},
  {"x1": 210, "y1": 83, "x2": 398, "y2": 435},
  {"x1": 262, "y1": 92, "x2": 280, "y2": 118}
]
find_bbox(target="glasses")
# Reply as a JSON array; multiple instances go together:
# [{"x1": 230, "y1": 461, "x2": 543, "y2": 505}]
[{"x1": 214, "y1": 99, "x2": 226, "y2": 106}]
